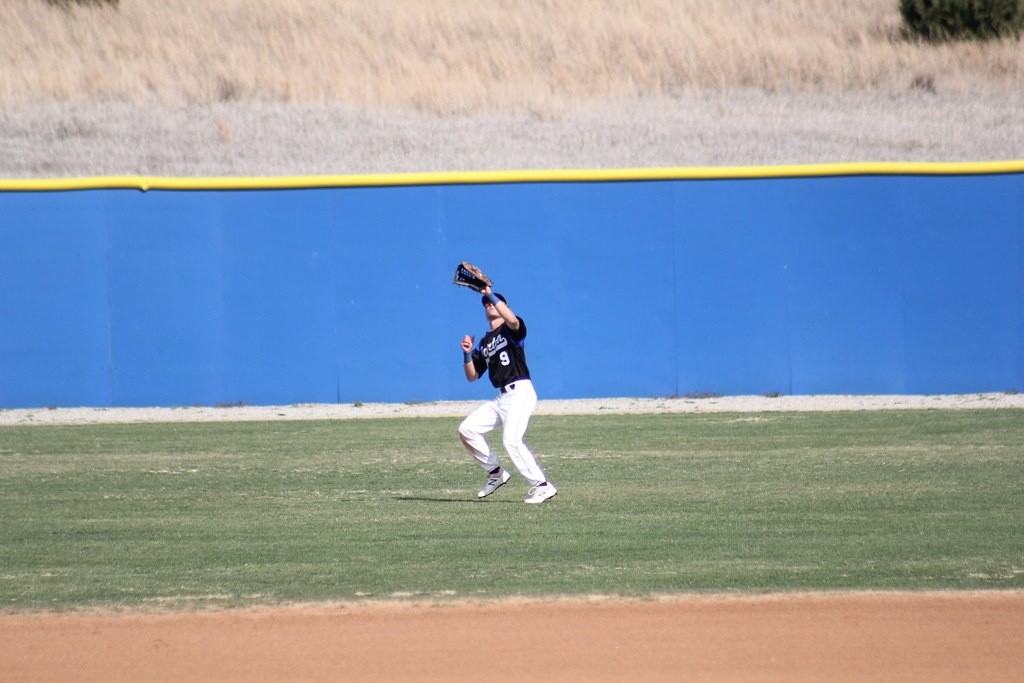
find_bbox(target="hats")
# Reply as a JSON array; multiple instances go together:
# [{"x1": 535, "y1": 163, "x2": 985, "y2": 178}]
[{"x1": 482, "y1": 293, "x2": 509, "y2": 309}]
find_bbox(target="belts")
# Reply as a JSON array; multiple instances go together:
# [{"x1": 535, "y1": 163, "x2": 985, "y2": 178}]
[{"x1": 501, "y1": 385, "x2": 515, "y2": 393}]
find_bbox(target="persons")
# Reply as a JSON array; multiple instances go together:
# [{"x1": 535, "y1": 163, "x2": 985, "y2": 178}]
[{"x1": 459, "y1": 286, "x2": 558, "y2": 503}]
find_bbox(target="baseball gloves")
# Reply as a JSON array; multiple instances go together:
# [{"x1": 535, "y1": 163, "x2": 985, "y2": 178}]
[{"x1": 453, "y1": 261, "x2": 492, "y2": 292}]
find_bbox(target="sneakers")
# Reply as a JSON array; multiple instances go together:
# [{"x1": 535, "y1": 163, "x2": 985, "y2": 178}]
[
  {"x1": 478, "y1": 467, "x2": 510, "y2": 498},
  {"x1": 525, "y1": 481, "x2": 557, "y2": 504}
]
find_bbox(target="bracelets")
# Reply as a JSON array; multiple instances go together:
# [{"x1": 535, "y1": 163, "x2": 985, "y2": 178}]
[
  {"x1": 487, "y1": 293, "x2": 500, "y2": 306},
  {"x1": 464, "y1": 352, "x2": 471, "y2": 363}
]
